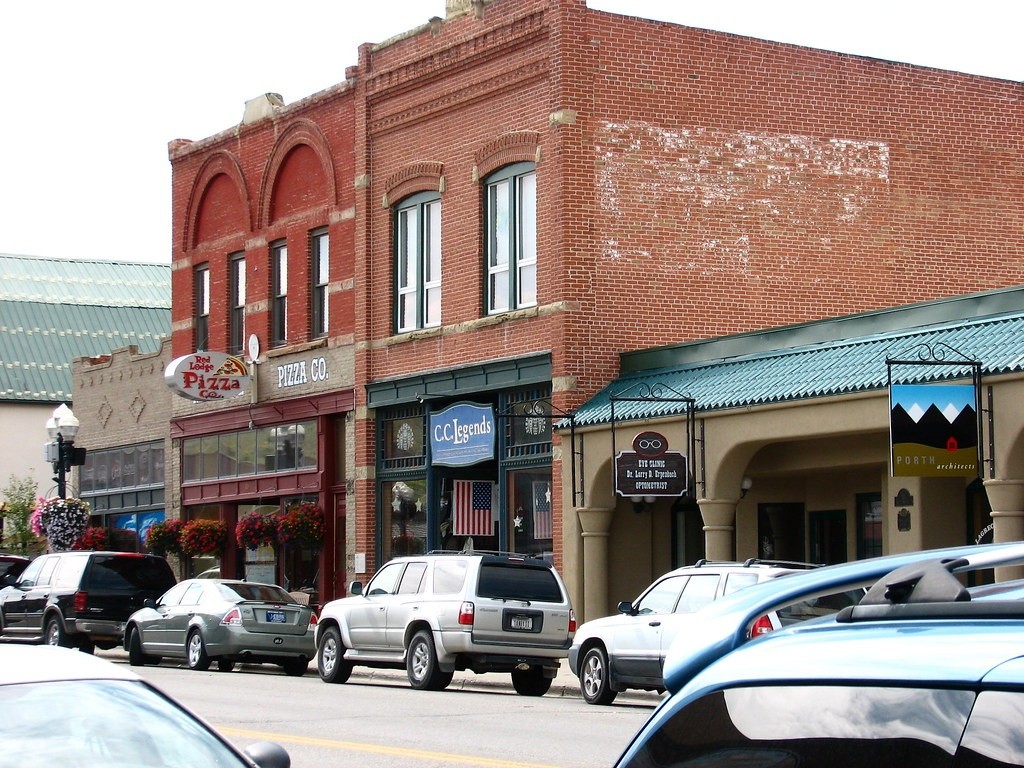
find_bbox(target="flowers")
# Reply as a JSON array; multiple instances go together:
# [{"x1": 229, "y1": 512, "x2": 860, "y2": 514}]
[
  {"x1": 276, "y1": 503, "x2": 327, "y2": 549},
  {"x1": 26, "y1": 497, "x2": 107, "y2": 551},
  {"x1": 235, "y1": 512, "x2": 279, "y2": 551},
  {"x1": 145, "y1": 519, "x2": 186, "y2": 557},
  {"x1": 176, "y1": 518, "x2": 229, "y2": 560}
]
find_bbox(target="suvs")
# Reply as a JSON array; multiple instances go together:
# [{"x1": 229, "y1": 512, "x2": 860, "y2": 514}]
[
  {"x1": 314, "y1": 550, "x2": 576, "y2": 698},
  {"x1": 611, "y1": 540, "x2": 1024, "y2": 768},
  {"x1": 197, "y1": 563, "x2": 303, "y2": 595},
  {"x1": 568, "y1": 557, "x2": 866, "y2": 705},
  {"x1": 0, "y1": 550, "x2": 179, "y2": 658}
]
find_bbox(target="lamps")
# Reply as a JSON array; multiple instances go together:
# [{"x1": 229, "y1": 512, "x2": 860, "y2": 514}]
[
  {"x1": 631, "y1": 496, "x2": 656, "y2": 513},
  {"x1": 740, "y1": 477, "x2": 753, "y2": 500}
]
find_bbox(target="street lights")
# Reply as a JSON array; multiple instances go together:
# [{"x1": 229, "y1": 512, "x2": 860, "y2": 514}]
[
  {"x1": 270, "y1": 424, "x2": 304, "y2": 470},
  {"x1": 47, "y1": 404, "x2": 79, "y2": 500}
]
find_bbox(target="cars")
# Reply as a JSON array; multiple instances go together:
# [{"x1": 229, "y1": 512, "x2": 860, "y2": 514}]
[
  {"x1": 0, "y1": 642, "x2": 290, "y2": 768},
  {"x1": 123, "y1": 578, "x2": 319, "y2": 676},
  {"x1": 0, "y1": 553, "x2": 32, "y2": 591}
]
